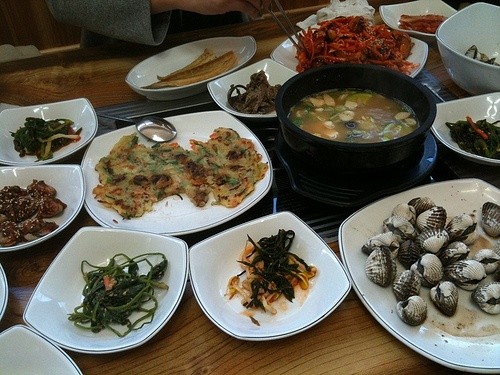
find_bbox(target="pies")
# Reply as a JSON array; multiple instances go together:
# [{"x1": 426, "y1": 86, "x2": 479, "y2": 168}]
[{"x1": 93, "y1": 127, "x2": 268, "y2": 221}]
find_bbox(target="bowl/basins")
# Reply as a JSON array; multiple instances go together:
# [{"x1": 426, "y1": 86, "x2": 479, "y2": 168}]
[
  {"x1": 436, "y1": 3, "x2": 500, "y2": 96},
  {"x1": 276, "y1": 65, "x2": 437, "y2": 176}
]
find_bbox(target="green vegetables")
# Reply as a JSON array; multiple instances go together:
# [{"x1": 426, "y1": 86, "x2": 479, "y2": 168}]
[
  {"x1": 445, "y1": 119, "x2": 500, "y2": 161},
  {"x1": 8, "y1": 117, "x2": 83, "y2": 162},
  {"x1": 294, "y1": 106, "x2": 403, "y2": 141},
  {"x1": 236, "y1": 229, "x2": 310, "y2": 326},
  {"x1": 67, "y1": 253, "x2": 169, "y2": 336}
]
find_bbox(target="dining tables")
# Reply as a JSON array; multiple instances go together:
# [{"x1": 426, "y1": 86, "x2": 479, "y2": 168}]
[{"x1": 0, "y1": 3, "x2": 500, "y2": 375}]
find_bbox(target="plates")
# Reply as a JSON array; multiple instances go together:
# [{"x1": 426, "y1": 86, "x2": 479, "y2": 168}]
[
  {"x1": 430, "y1": 92, "x2": 500, "y2": 166},
  {"x1": 125, "y1": 35, "x2": 257, "y2": 101},
  {"x1": 81, "y1": 110, "x2": 274, "y2": 236},
  {"x1": 22, "y1": 226, "x2": 190, "y2": 355},
  {"x1": 0, "y1": 264, "x2": 9, "y2": 320},
  {"x1": 188, "y1": 211, "x2": 352, "y2": 341},
  {"x1": 0, "y1": 165, "x2": 86, "y2": 252},
  {"x1": 276, "y1": 129, "x2": 438, "y2": 208},
  {"x1": 0, "y1": 324, "x2": 83, "y2": 375},
  {"x1": 270, "y1": 18, "x2": 429, "y2": 78},
  {"x1": 207, "y1": 59, "x2": 298, "y2": 118},
  {"x1": 0, "y1": 98, "x2": 98, "y2": 166},
  {"x1": 338, "y1": 177, "x2": 500, "y2": 373},
  {"x1": 379, "y1": 0, "x2": 458, "y2": 41}
]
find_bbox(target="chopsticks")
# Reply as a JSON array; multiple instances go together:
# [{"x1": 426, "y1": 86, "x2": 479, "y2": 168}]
[{"x1": 265, "y1": 0, "x2": 311, "y2": 60}]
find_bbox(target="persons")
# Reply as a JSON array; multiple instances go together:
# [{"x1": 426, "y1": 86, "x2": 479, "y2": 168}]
[{"x1": 48, "y1": 0, "x2": 273, "y2": 47}]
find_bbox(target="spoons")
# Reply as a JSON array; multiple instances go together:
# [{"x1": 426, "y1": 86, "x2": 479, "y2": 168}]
[{"x1": 97, "y1": 113, "x2": 177, "y2": 142}]
